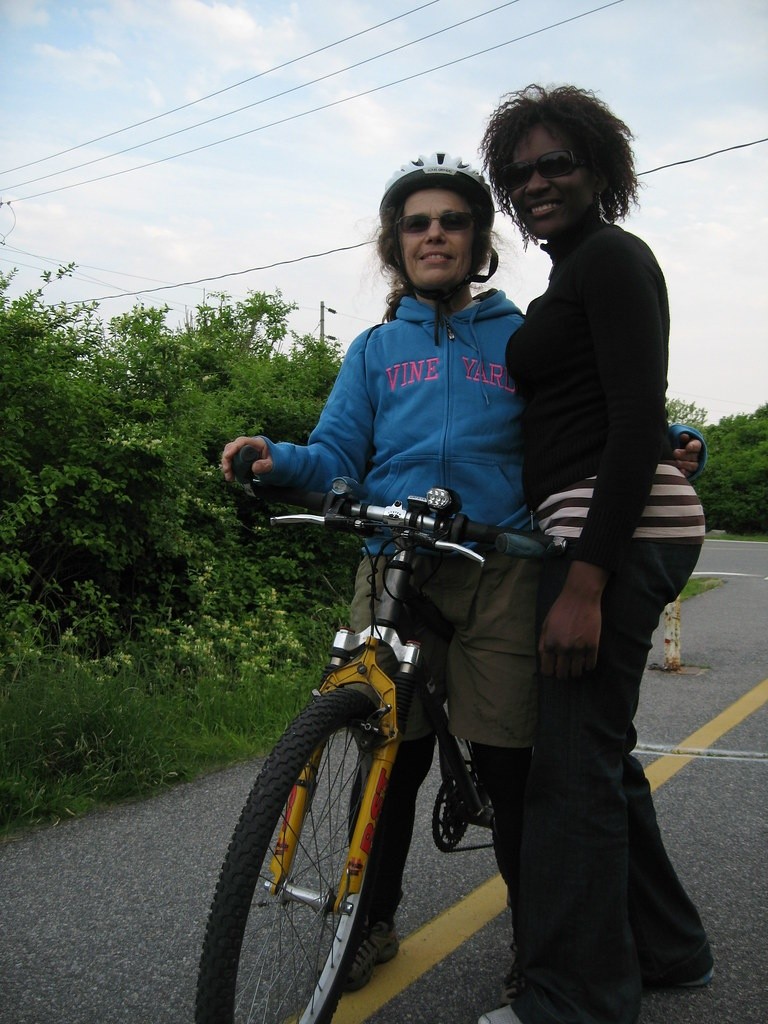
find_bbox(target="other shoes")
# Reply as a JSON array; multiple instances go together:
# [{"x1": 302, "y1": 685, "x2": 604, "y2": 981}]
[
  {"x1": 661, "y1": 961, "x2": 715, "y2": 988},
  {"x1": 479, "y1": 1004, "x2": 525, "y2": 1024},
  {"x1": 314, "y1": 917, "x2": 404, "y2": 988},
  {"x1": 500, "y1": 959, "x2": 526, "y2": 1006}
]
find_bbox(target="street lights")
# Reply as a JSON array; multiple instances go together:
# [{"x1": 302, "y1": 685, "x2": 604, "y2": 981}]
[{"x1": 319, "y1": 300, "x2": 339, "y2": 350}]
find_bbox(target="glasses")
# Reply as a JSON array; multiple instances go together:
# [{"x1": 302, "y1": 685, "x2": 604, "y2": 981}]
[
  {"x1": 394, "y1": 211, "x2": 478, "y2": 235},
  {"x1": 498, "y1": 147, "x2": 595, "y2": 191}
]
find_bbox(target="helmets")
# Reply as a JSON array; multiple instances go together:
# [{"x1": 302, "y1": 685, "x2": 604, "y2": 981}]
[{"x1": 378, "y1": 151, "x2": 497, "y2": 232}]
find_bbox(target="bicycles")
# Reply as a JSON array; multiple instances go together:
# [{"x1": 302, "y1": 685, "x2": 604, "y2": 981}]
[{"x1": 192, "y1": 444, "x2": 570, "y2": 1024}]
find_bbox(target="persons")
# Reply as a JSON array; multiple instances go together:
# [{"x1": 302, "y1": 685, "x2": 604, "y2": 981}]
[
  {"x1": 472, "y1": 83, "x2": 722, "y2": 1024},
  {"x1": 213, "y1": 148, "x2": 711, "y2": 1005}
]
